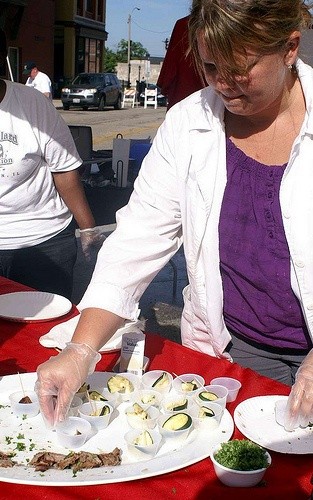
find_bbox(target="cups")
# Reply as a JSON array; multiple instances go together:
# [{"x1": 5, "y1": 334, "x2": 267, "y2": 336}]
[{"x1": 8, "y1": 370, "x2": 242, "y2": 460}]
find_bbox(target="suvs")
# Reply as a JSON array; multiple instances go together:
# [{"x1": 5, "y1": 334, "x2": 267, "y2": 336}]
[{"x1": 61, "y1": 72, "x2": 124, "y2": 112}]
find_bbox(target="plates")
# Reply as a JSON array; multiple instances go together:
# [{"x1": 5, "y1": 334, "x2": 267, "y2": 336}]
[
  {"x1": 50, "y1": 321, "x2": 144, "y2": 353},
  {"x1": 0, "y1": 374, "x2": 234, "y2": 486},
  {"x1": 113, "y1": 356, "x2": 149, "y2": 375},
  {"x1": 234, "y1": 395, "x2": 313, "y2": 455},
  {"x1": 0, "y1": 291, "x2": 72, "y2": 322}
]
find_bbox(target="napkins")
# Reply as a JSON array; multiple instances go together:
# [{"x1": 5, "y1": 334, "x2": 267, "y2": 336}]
[{"x1": 39, "y1": 314, "x2": 149, "y2": 351}]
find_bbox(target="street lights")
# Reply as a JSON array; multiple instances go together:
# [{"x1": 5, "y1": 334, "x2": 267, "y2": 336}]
[{"x1": 127, "y1": 6, "x2": 140, "y2": 87}]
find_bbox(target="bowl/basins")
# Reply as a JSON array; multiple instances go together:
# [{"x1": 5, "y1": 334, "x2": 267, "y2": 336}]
[
  {"x1": 275, "y1": 400, "x2": 300, "y2": 428},
  {"x1": 210, "y1": 441, "x2": 271, "y2": 488}
]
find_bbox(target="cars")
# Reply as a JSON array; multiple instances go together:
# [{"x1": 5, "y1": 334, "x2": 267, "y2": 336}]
[{"x1": 139, "y1": 85, "x2": 168, "y2": 107}]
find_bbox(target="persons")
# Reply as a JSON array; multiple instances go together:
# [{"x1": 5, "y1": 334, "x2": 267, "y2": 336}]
[
  {"x1": 22, "y1": 60, "x2": 52, "y2": 102},
  {"x1": 141, "y1": 76, "x2": 146, "y2": 83},
  {"x1": 0, "y1": 28, "x2": 107, "y2": 299},
  {"x1": 34, "y1": 0, "x2": 313, "y2": 428}
]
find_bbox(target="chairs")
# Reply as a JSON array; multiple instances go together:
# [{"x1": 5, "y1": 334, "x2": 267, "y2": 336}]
[
  {"x1": 122, "y1": 87, "x2": 140, "y2": 108},
  {"x1": 144, "y1": 88, "x2": 158, "y2": 109}
]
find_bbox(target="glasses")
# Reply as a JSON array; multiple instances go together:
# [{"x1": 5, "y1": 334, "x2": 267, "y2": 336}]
[{"x1": 199, "y1": 53, "x2": 264, "y2": 82}]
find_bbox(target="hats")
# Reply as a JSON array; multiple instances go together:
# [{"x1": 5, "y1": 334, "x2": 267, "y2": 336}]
[{"x1": 22, "y1": 59, "x2": 37, "y2": 74}]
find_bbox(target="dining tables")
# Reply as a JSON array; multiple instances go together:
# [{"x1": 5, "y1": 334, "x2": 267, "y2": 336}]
[{"x1": 0, "y1": 272, "x2": 313, "y2": 500}]
